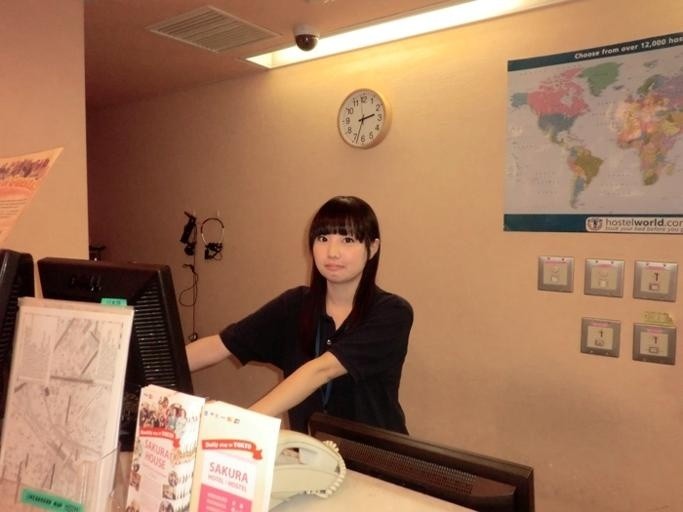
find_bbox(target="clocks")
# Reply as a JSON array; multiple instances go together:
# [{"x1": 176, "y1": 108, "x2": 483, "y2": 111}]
[{"x1": 336, "y1": 89, "x2": 392, "y2": 150}]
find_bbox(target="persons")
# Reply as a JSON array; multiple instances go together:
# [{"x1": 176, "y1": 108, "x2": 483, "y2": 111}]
[{"x1": 185, "y1": 195, "x2": 414, "y2": 434}]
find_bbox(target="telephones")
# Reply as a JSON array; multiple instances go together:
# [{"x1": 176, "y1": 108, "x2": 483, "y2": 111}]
[{"x1": 269, "y1": 429, "x2": 341, "y2": 512}]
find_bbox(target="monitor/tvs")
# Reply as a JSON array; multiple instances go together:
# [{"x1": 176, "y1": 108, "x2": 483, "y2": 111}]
[
  {"x1": 37, "y1": 256, "x2": 194, "y2": 452},
  {"x1": 0, "y1": 248, "x2": 35, "y2": 420}
]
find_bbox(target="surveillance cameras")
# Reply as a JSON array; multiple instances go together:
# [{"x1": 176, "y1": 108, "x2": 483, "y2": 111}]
[{"x1": 292, "y1": 24, "x2": 320, "y2": 52}]
